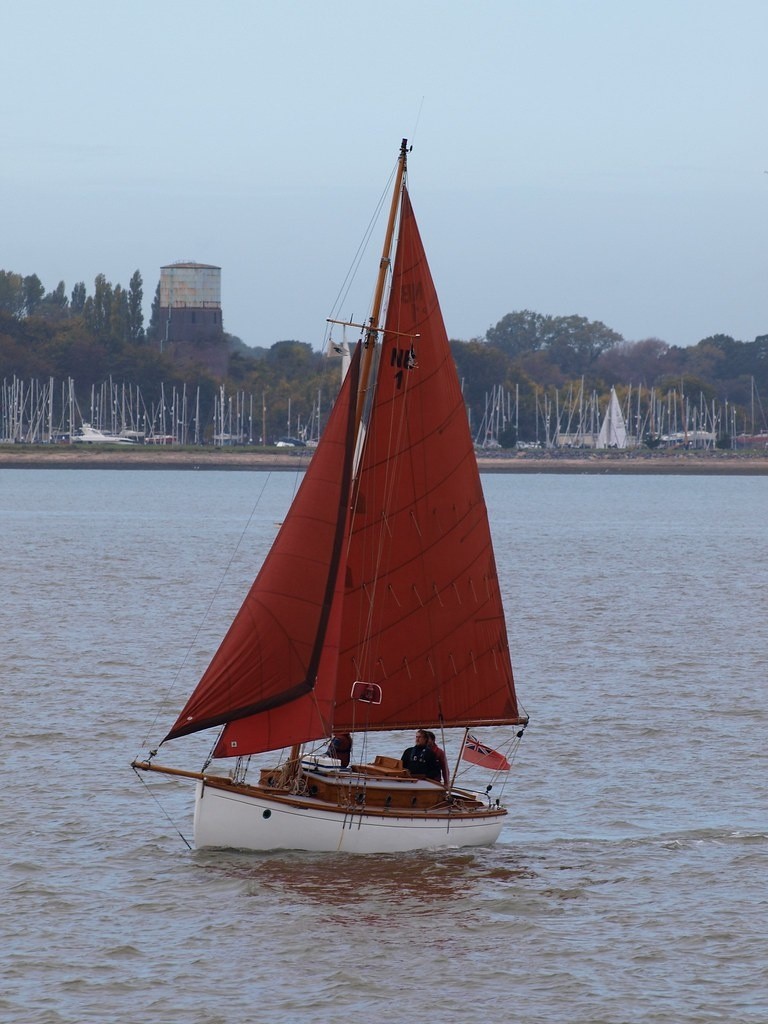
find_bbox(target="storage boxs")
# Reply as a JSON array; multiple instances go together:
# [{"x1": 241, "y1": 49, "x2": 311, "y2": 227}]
[{"x1": 300, "y1": 753, "x2": 341, "y2": 774}]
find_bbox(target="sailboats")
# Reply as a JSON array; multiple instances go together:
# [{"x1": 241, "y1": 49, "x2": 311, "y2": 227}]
[
  {"x1": 0, "y1": 372, "x2": 768, "y2": 452},
  {"x1": 125, "y1": 136, "x2": 533, "y2": 856}
]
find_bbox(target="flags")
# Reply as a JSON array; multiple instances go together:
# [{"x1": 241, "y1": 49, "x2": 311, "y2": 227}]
[{"x1": 462, "y1": 733, "x2": 511, "y2": 771}]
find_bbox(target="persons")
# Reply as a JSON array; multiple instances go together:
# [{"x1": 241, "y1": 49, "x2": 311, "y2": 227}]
[
  {"x1": 323, "y1": 731, "x2": 353, "y2": 769},
  {"x1": 400, "y1": 730, "x2": 450, "y2": 790}
]
